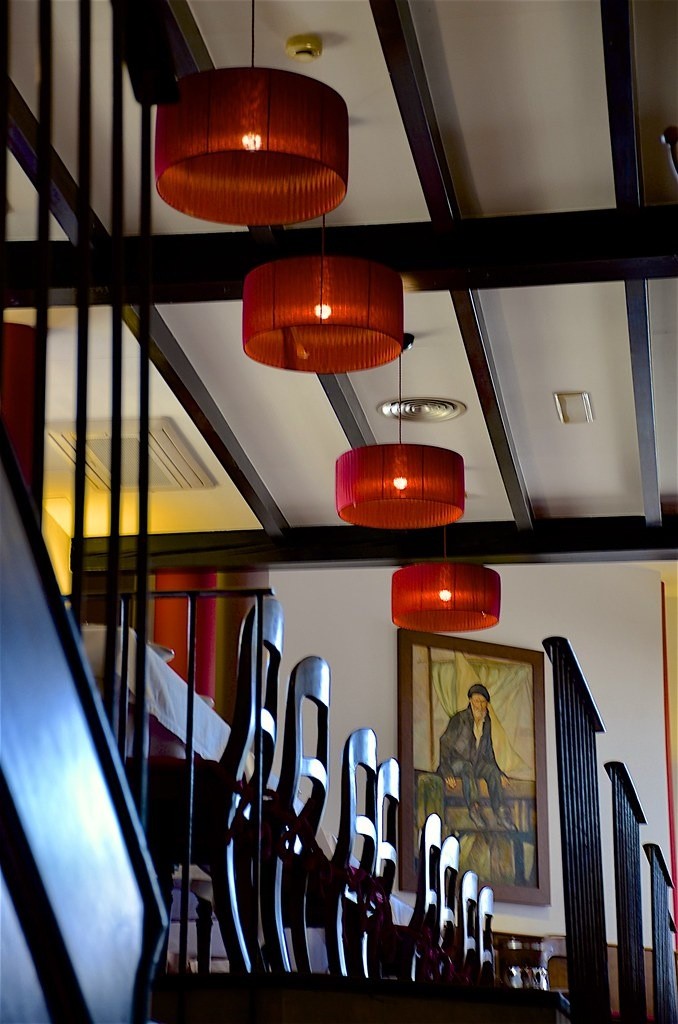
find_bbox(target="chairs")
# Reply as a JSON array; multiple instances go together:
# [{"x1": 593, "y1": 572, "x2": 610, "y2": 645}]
[{"x1": 125, "y1": 598, "x2": 497, "y2": 986}]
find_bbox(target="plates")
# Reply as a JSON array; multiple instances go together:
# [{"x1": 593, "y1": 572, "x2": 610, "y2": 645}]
[
  {"x1": 198, "y1": 693, "x2": 216, "y2": 709},
  {"x1": 148, "y1": 640, "x2": 174, "y2": 661}
]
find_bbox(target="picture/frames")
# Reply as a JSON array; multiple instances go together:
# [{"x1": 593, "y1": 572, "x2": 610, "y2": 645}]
[{"x1": 398, "y1": 628, "x2": 551, "y2": 906}]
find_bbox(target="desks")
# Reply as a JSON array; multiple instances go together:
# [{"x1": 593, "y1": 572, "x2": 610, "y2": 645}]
[{"x1": 83, "y1": 622, "x2": 414, "y2": 926}]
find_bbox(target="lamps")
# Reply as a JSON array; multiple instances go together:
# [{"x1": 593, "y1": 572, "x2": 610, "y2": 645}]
[
  {"x1": 242, "y1": 213, "x2": 404, "y2": 373},
  {"x1": 154, "y1": 0, "x2": 350, "y2": 225},
  {"x1": 336, "y1": 334, "x2": 466, "y2": 530},
  {"x1": 392, "y1": 525, "x2": 501, "y2": 633}
]
